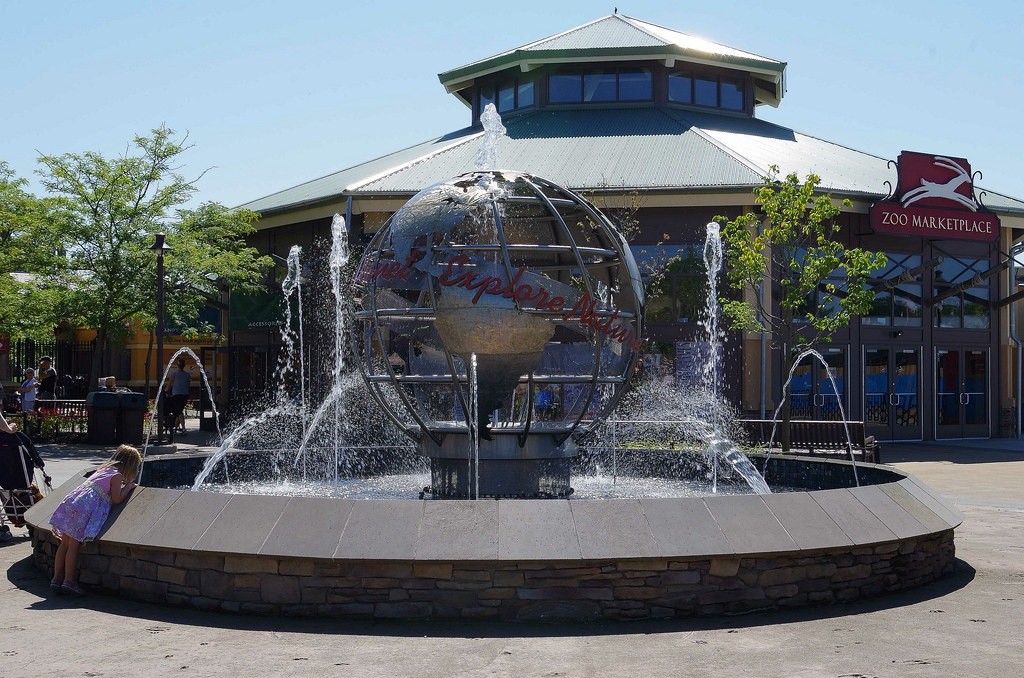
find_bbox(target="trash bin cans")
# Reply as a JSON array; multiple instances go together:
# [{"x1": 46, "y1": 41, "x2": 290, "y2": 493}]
[{"x1": 85, "y1": 392, "x2": 148, "y2": 449}]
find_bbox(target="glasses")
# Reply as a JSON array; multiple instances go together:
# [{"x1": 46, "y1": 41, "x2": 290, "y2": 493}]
[{"x1": 27, "y1": 371, "x2": 34, "y2": 374}]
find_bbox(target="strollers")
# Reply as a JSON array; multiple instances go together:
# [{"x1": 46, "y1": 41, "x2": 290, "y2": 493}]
[{"x1": 0, "y1": 430, "x2": 53, "y2": 544}]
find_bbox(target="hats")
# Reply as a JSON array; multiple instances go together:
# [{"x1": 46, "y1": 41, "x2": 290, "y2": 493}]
[{"x1": 38, "y1": 356, "x2": 51, "y2": 362}]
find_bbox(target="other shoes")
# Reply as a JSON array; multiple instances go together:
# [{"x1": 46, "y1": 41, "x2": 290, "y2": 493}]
[
  {"x1": 0, "y1": 530, "x2": 13, "y2": 542},
  {"x1": 182, "y1": 429, "x2": 188, "y2": 435},
  {"x1": 50, "y1": 577, "x2": 64, "y2": 594},
  {"x1": 174, "y1": 430, "x2": 178, "y2": 435},
  {"x1": 62, "y1": 579, "x2": 86, "y2": 597}
]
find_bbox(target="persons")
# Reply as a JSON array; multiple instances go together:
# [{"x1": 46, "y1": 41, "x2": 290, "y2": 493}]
[
  {"x1": 423, "y1": 348, "x2": 677, "y2": 423},
  {"x1": 17, "y1": 357, "x2": 58, "y2": 412},
  {"x1": 104, "y1": 376, "x2": 118, "y2": 393},
  {"x1": 48, "y1": 443, "x2": 141, "y2": 595},
  {"x1": 163, "y1": 359, "x2": 191, "y2": 435},
  {"x1": 0, "y1": 413, "x2": 18, "y2": 434}
]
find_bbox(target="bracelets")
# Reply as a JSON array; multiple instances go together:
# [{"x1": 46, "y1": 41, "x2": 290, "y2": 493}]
[{"x1": 164, "y1": 389, "x2": 167, "y2": 392}]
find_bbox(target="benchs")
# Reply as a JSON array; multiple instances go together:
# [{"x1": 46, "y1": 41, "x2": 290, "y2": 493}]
[{"x1": 580, "y1": 419, "x2": 874, "y2": 462}]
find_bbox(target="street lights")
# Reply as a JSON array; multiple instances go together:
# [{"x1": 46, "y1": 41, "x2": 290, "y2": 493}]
[{"x1": 148, "y1": 232, "x2": 173, "y2": 445}]
